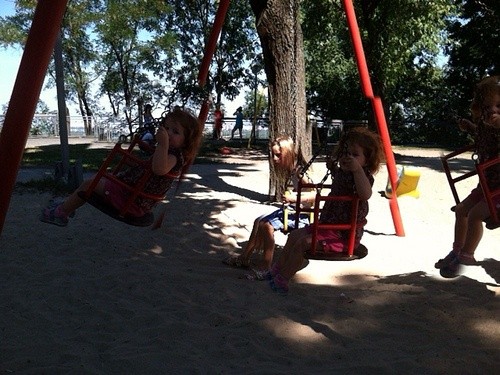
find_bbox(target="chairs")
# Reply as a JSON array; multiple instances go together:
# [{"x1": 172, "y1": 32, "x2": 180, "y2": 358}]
[
  {"x1": 82, "y1": 138, "x2": 183, "y2": 228},
  {"x1": 295, "y1": 183, "x2": 368, "y2": 261},
  {"x1": 441, "y1": 142, "x2": 500, "y2": 230}
]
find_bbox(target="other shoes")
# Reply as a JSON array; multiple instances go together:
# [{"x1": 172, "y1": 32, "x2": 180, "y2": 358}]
[
  {"x1": 268, "y1": 265, "x2": 289, "y2": 292},
  {"x1": 436, "y1": 251, "x2": 463, "y2": 278}
]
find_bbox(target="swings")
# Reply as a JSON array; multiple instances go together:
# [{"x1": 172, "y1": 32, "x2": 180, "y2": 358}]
[
  {"x1": 279, "y1": 0, "x2": 321, "y2": 234},
  {"x1": 290, "y1": 1, "x2": 369, "y2": 262},
  {"x1": 438, "y1": 144, "x2": 500, "y2": 229},
  {"x1": 76, "y1": 0, "x2": 235, "y2": 229}
]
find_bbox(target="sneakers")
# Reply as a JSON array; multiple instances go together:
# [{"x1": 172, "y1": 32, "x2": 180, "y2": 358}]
[
  {"x1": 40, "y1": 207, "x2": 69, "y2": 226},
  {"x1": 51, "y1": 197, "x2": 76, "y2": 219}
]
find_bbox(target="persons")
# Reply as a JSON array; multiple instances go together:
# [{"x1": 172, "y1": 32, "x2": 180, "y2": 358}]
[
  {"x1": 434, "y1": 75, "x2": 500, "y2": 278},
  {"x1": 35, "y1": 107, "x2": 202, "y2": 226},
  {"x1": 255, "y1": 127, "x2": 384, "y2": 297},
  {"x1": 142, "y1": 104, "x2": 243, "y2": 142},
  {"x1": 222, "y1": 136, "x2": 319, "y2": 281}
]
